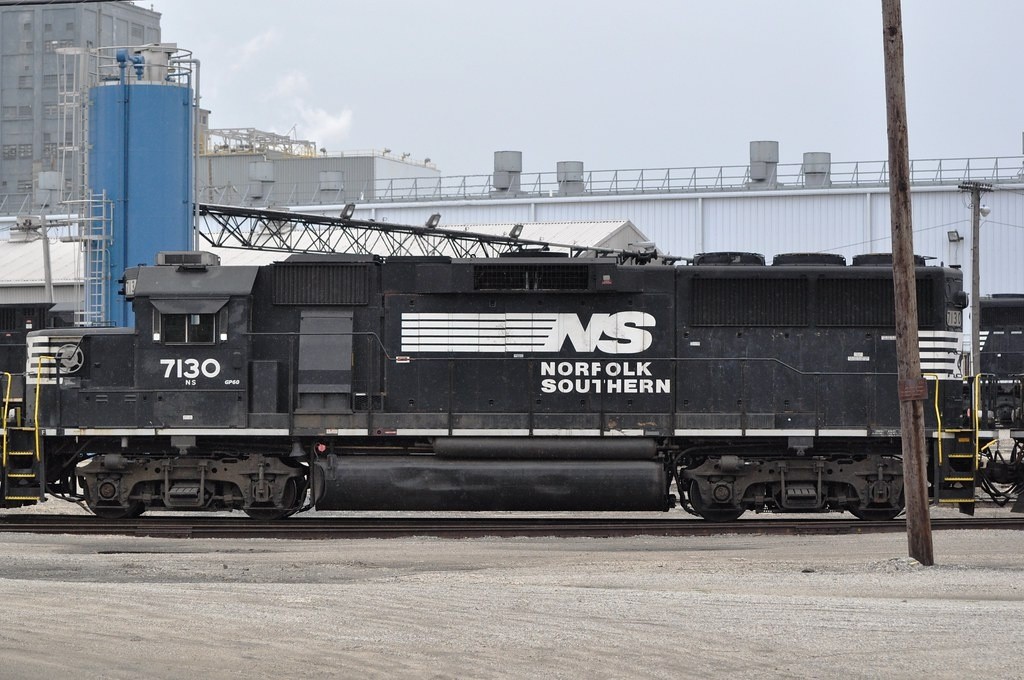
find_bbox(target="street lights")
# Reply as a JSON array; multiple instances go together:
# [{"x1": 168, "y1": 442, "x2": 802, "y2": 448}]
[{"x1": 957, "y1": 179, "x2": 997, "y2": 447}]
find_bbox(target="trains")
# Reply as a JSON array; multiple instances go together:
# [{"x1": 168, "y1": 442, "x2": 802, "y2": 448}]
[{"x1": 1, "y1": 249, "x2": 987, "y2": 521}]
